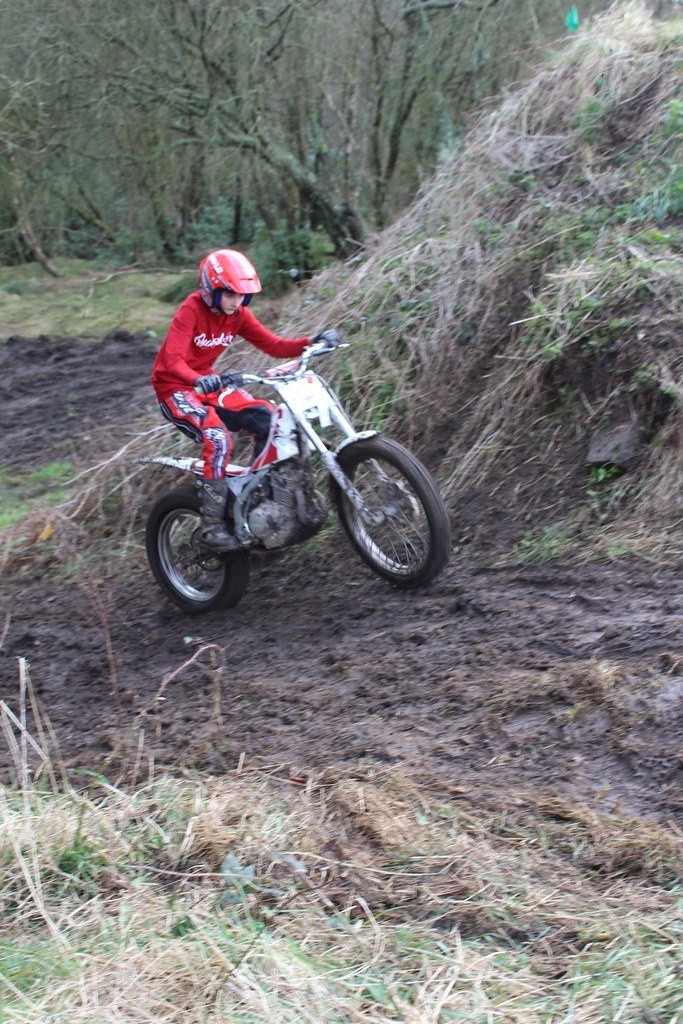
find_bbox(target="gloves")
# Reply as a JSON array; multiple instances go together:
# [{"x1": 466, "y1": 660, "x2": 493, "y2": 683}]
[
  {"x1": 311, "y1": 328, "x2": 341, "y2": 348},
  {"x1": 195, "y1": 374, "x2": 224, "y2": 394}
]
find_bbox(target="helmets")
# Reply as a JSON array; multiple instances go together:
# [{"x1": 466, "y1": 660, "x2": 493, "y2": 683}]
[{"x1": 199, "y1": 249, "x2": 262, "y2": 308}]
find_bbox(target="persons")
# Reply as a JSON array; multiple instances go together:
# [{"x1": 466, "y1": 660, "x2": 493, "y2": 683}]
[{"x1": 150, "y1": 248, "x2": 341, "y2": 553}]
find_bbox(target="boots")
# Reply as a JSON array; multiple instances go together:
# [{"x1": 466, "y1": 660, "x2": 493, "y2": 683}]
[{"x1": 200, "y1": 477, "x2": 240, "y2": 551}]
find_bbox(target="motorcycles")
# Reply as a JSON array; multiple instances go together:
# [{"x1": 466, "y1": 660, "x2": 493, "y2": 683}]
[{"x1": 132, "y1": 342, "x2": 451, "y2": 616}]
